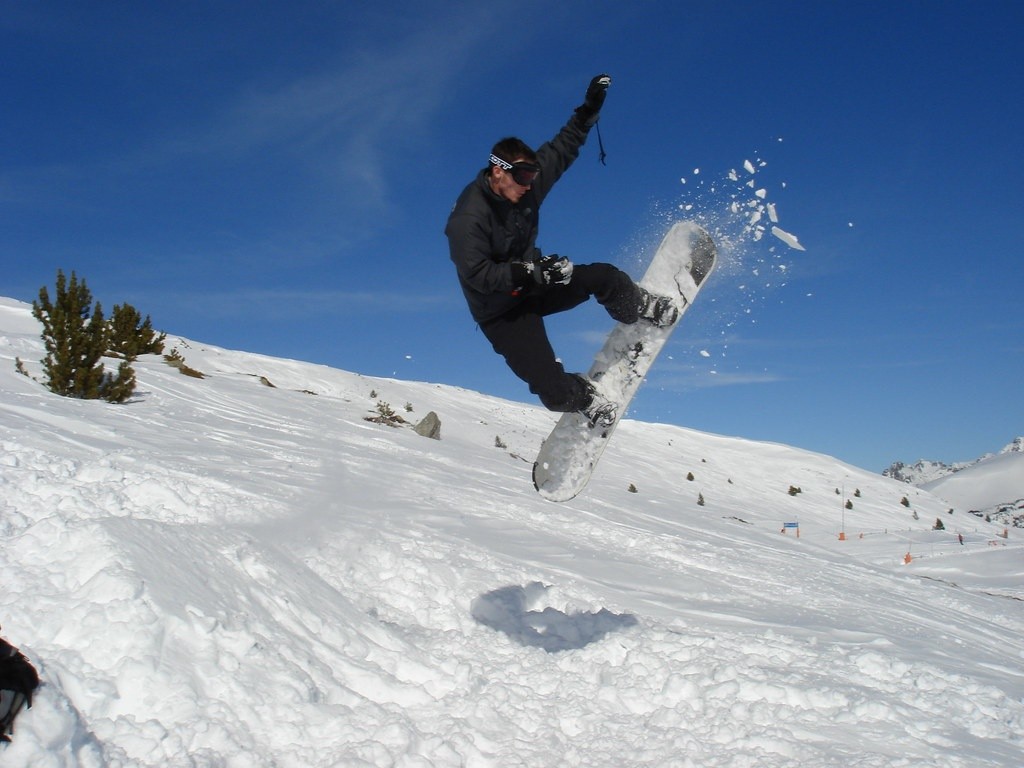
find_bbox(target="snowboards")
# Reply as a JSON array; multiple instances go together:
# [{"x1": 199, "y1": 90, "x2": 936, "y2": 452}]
[{"x1": 530, "y1": 221, "x2": 717, "y2": 504}]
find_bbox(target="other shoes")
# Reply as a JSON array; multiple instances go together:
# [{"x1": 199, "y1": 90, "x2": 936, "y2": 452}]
[
  {"x1": 637, "y1": 287, "x2": 678, "y2": 327},
  {"x1": 574, "y1": 372, "x2": 617, "y2": 429}
]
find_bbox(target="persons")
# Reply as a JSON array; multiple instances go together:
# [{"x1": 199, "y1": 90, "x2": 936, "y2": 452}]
[{"x1": 444, "y1": 72, "x2": 678, "y2": 430}]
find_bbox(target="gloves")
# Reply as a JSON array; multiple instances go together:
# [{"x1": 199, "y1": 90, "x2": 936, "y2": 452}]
[
  {"x1": 510, "y1": 253, "x2": 578, "y2": 295},
  {"x1": 583, "y1": 73, "x2": 612, "y2": 112}
]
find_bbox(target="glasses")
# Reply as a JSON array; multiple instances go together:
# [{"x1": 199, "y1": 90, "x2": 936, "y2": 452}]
[{"x1": 488, "y1": 153, "x2": 542, "y2": 186}]
